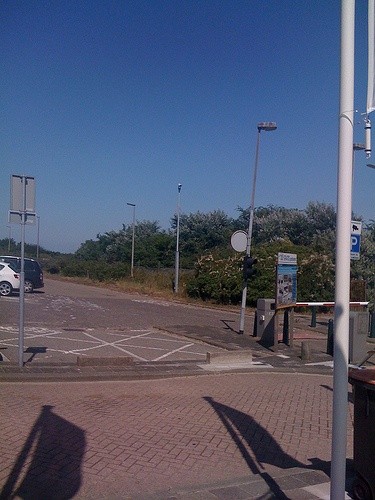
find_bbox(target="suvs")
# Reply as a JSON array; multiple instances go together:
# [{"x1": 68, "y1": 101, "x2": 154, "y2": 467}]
[{"x1": 0, "y1": 256, "x2": 44, "y2": 293}]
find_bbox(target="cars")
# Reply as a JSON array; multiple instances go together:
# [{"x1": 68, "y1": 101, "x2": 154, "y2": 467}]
[{"x1": 0, "y1": 262, "x2": 21, "y2": 296}]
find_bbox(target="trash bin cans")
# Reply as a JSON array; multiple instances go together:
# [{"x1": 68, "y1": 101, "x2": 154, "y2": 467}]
[
  {"x1": 347, "y1": 365, "x2": 374, "y2": 500},
  {"x1": 255, "y1": 298, "x2": 285, "y2": 338}
]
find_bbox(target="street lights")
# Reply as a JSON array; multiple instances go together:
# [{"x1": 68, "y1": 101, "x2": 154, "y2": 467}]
[
  {"x1": 127, "y1": 202, "x2": 136, "y2": 278},
  {"x1": 238, "y1": 121, "x2": 278, "y2": 334},
  {"x1": 173, "y1": 184, "x2": 183, "y2": 295}
]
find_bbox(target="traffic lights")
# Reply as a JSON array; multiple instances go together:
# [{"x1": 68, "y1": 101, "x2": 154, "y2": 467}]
[{"x1": 242, "y1": 257, "x2": 258, "y2": 278}]
